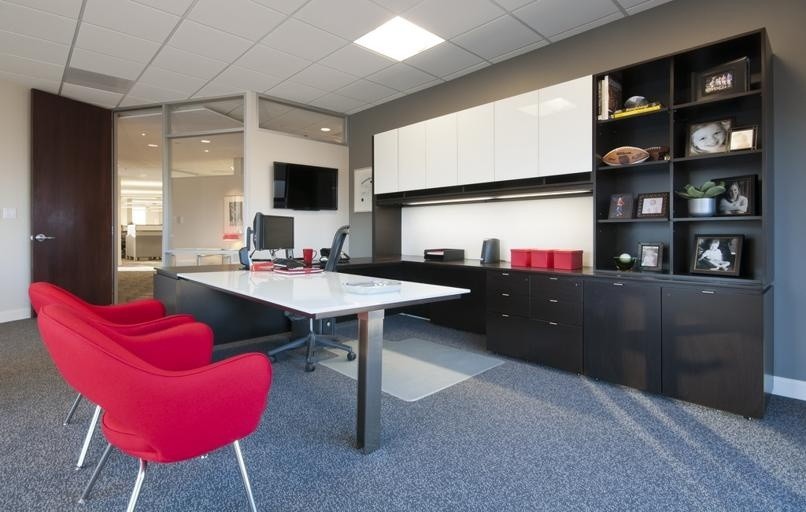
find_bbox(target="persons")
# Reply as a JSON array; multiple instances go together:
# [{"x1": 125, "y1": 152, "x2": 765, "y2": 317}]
[
  {"x1": 709, "y1": 73, "x2": 733, "y2": 90},
  {"x1": 720, "y1": 181, "x2": 748, "y2": 215},
  {"x1": 689, "y1": 122, "x2": 728, "y2": 153},
  {"x1": 698, "y1": 240, "x2": 730, "y2": 271},
  {"x1": 616, "y1": 195, "x2": 624, "y2": 216}
]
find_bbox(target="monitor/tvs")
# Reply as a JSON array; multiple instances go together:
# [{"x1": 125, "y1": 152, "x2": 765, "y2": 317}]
[
  {"x1": 263, "y1": 215, "x2": 294, "y2": 249},
  {"x1": 255, "y1": 213, "x2": 264, "y2": 251},
  {"x1": 271, "y1": 161, "x2": 338, "y2": 210}
]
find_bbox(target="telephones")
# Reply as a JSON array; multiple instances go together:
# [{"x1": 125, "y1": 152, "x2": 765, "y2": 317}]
[{"x1": 320, "y1": 249, "x2": 350, "y2": 264}]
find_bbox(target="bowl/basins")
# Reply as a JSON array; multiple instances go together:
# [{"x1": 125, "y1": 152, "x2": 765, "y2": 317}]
[{"x1": 612, "y1": 256, "x2": 639, "y2": 270}]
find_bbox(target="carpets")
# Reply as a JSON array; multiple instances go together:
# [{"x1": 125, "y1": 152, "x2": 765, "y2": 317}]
[{"x1": 318, "y1": 337, "x2": 505, "y2": 403}]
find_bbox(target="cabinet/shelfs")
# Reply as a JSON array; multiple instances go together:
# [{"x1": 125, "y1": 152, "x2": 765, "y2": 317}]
[
  {"x1": 671, "y1": 39, "x2": 776, "y2": 283},
  {"x1": 372, "y1": 73, "x2": 593, "y2": 200},
  {"x1": 532, "y1": 266, "x2": 584, "y2": 374},
  {"x1": 663, "y1": 281, "x2": 774, "y2": 420},
  {"x1": 594, "y1": 52, "x2": 672, "y2": 278},
  {"x1": 485, "y1": 268, "x2": 532, "y2": 365},
  {"x1": 585, "y1": 274, "x2": 662, "y2": 398}
]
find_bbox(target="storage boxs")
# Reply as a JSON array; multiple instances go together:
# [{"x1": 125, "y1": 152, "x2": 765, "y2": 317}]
[
  {"x1": 510, "y1": 248, "x2": 531, "y2": 268},
  {"x1": 554, "y1": 249, "x2": 584, "y2": 272},
  {"x1": 530, "y1": 249, "x2": 558, "y2": 269}
]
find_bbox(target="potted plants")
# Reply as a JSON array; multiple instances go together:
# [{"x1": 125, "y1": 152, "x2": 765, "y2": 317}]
[{"x1": 676, "y1": 182, "x2": 727, "y2": 216}]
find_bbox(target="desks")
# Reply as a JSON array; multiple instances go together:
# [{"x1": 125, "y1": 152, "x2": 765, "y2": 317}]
[
  {"x1": 317, "y1": 256, "x2": 511, "y2": 335},
  {"x1": 154, "y1": 264, "x2": 473, "y2": 453}
]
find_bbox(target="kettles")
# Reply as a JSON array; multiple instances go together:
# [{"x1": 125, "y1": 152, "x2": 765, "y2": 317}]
[{"x1": 480, "y1": 238, "x2": 501, "y2": 262}]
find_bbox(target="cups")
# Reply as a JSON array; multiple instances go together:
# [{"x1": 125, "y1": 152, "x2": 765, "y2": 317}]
[{"x1": 303, "y1": 249, "x2": 317, "y2": 265}]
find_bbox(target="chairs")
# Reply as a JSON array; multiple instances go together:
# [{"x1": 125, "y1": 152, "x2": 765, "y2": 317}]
[
  {"x1": 165, "y1": 248, "x2": 240, "y2": 266},
  {"x1": 264, "y1": 223, "x2": 355, "y2": 371},
  {"x1": 34, "y1": 303, "x2": 273, "y2": 511},
  {"x1": 25, "y1": 278, "x2": 196, "y2": 470}
]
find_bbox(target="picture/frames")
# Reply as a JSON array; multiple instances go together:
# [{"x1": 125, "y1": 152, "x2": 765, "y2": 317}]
[
  {"x1": 688, "y1": 59, "x2": 751, "y2": 103},
  {"x1": 637, "y1": 240, "x2": 664, "y2": 273},
  {"x1": 724, "y1": 123, "x2": 758, "y2": 153},
  {"x1": 711, "y1": 175, "x2": 759, "y2": 217},
  {"x1": 636, "y1": 191, "x2": 669, "y2": 217},
  {"x1": 683, "y1": 114, "x2": 735, "y2": 156},
  {"x1": 689, "y1": 232, "x2": 744, "y2": 277}
]
